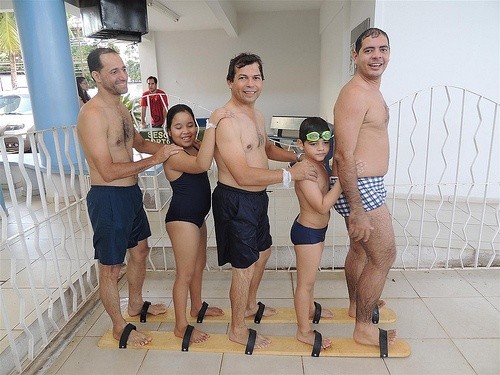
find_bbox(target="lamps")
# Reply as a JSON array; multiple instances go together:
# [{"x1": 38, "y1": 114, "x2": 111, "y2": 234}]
[{"x1": 147, "y1": 0, "x2": 180, "y2": 23}]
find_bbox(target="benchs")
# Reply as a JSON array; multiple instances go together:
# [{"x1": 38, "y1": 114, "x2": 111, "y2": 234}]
[
  {"x1": 132, "y1": 147, "x2": 173, "y2": 212},
  {"x1": 267, "y1": 114, "x2": 334, "y2": 176},
  {"x1": 0, "y1": 152, "x2": 43, "y2": 198}
]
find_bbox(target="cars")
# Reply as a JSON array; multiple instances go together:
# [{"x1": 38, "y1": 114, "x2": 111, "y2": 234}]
[{"x1": 0, "y1": 88, "x2": 36, "y2": 151}]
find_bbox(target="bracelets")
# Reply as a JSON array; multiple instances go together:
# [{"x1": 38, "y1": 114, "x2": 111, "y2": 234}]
[
  {"x1": 206, "y1": 119, "x2": 216, "y2": 130},
  {"x1": 282, "y1": 168, "x2": 292, "y2": 187},
  {"x1": 297, "y1": 153, "x2": 304, "y2": 158}
]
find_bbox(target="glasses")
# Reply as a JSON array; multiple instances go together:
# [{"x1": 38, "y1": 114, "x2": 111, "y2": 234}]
[{"x1": 301, "y1": 130, "x2": 333, "y2": 142}]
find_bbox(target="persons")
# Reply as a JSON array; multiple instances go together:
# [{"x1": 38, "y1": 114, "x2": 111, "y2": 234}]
[
  {"x1": 77, "y1": 48, "x2": 184, "y2": 350},
  {"x1": 75, "y1": 77, "x2": 92, "y2": 109},
  {"x1": 212, "y1": 52, "x2": 318, "y2": 349},
  {"x1": 163, "y1": 104, "x2": 234, "y2": 344},
  {"x1": 140, "y1": 76, "x2": 169, "y2": 129},
  {"x1": 331, "y1": 29, "x2": 398, "y2": 346},
  {"x1": 289, "y1": 117, "x2": 367, "y2": 350}
]
